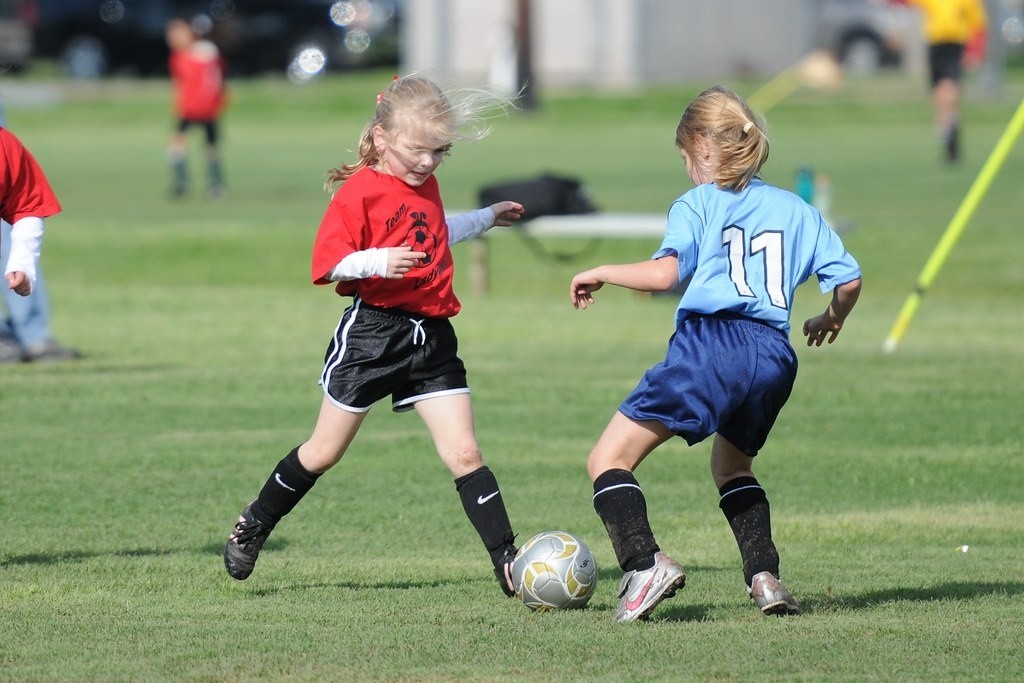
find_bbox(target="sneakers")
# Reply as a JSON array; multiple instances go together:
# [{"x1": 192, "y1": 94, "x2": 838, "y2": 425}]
[
  {"x1": 224, "y1": 497, "x2": 275, "y2": 580},
  {"x1": 747, "y1": 571, "x2": 798, "y2": 615},
  {"x1": 493, "y1": 529, "x2": 519, "y2": 598},
  {"x1": 614, "y1": 552, "x2": 686, "y2": 624}
]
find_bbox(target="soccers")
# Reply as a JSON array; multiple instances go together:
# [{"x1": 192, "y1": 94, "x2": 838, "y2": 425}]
[{"x1": 512, "y1": 531, "x2": 598, "y2": 611}]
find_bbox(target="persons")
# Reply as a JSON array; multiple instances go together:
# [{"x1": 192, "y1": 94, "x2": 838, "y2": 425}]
[
  {"x1": 572, "y1": 85, "x2": 862, "y2": 623},
  {"x1": 907, "y1": 0, "x2": 987, "y2": 163},
  {"x1": 164, "y1": 19, "x2": 232, "y2": 199},
  {"x1": 0, "y1": 128, "x2": 63, "y2": 362},
  {"x1": 224, "y1": 77, "x2": 520, "y2": 597}
]
